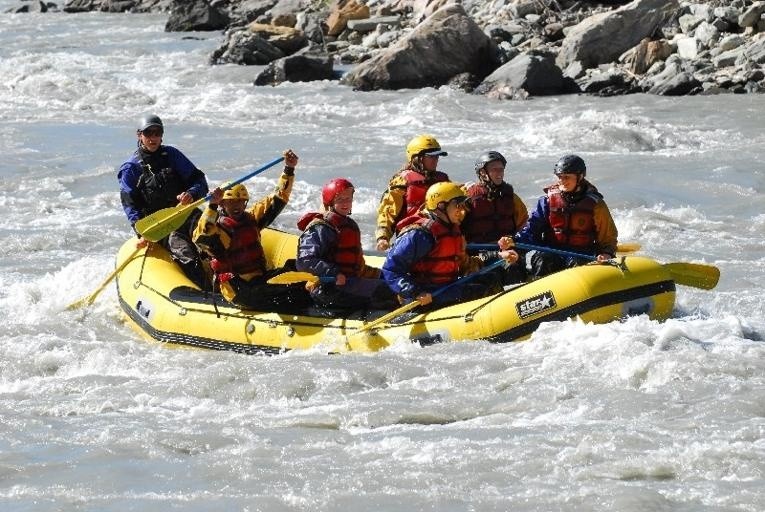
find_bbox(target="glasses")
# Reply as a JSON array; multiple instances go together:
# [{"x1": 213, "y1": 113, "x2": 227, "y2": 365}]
[{"x1": 143, "y1": 130, "x2": 162, "y2": 137}]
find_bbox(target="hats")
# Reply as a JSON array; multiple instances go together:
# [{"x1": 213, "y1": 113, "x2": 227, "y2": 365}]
[{"x1": 424, "y1": 150, "x2": 447, "y2": 157}]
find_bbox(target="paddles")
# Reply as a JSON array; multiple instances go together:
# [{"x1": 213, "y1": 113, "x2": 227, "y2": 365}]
[
  {"x1": 135, "y1": 155, "x2": 287, "y2": 242},
  {"x1": 511, "y1": 242, "x2": 720, "y2": 289},
  {"x1": 343, "y1": 257, "x2": 508, "y2": 334},
  {"x1": 64, "y1": 248, "x2": 139, "y2": 313},
  {"x1": 266, "y1": 270, "x2": 386, "y2": 285}
]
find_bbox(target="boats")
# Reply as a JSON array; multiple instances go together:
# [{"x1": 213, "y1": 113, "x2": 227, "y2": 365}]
[{"x1": 107, "y1": 222, "x2": 676, "y2": 360}]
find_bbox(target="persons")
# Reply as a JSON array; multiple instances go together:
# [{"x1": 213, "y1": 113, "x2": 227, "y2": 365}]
[
  {"x1": 459, "y1": 151, "x2": 530, "y2": 285},
  {"x1": 117, "y1": 114, "x2": 213, "y2": 292},
  {"x1": 497, "y1": 154, "x2": 619, "y2": 277},
  {"x1": 296, "y1": 177, "x2": 400, "y2": 319},
  {"x1": 191, "y1": 149, "x2": 315, "y2": 313},
  {"x1": 381, "y1": 181, "x2": 519, "y2": 314},
  {"x1": 375, "y1": 136, "x2": 465, "y2": 252}
]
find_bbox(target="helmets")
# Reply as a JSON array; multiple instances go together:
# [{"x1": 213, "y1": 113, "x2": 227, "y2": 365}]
[
  {"x1": 474, "y1": 151, "x2": 507, "y2": 177},
  {"x1": 426, "y1": 182, "x2": 472, "y2": 211},
  {"x1": 137, "y1": 114, "x2": 163, "y2": 131},
  {"x1": 220, "y1": 181, "x2": 249, "y2": 200},
  {"x1": 322, "y1": 178, "x2": 354, "y2": 205},
  {"x1": 553, "y1": 155, "x2": 586, "y2": 175},
  {"x1": 407, "y1": 134, "x2": 448, "y2": 163}
]
